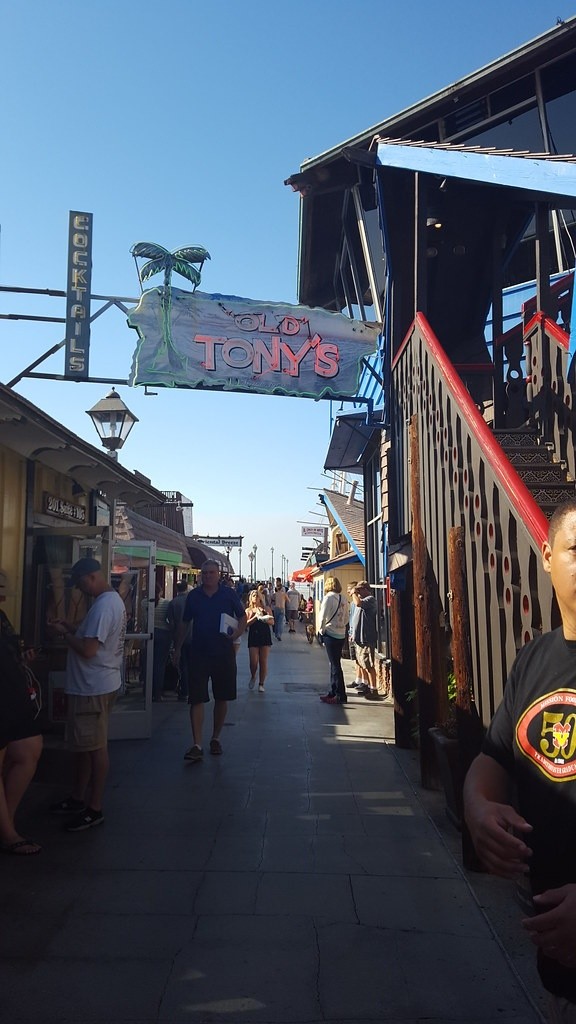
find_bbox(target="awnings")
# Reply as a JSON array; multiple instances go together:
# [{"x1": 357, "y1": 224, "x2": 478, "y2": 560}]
[{"x1": 187, "y1": 546, "x2": 234, "y2": 573}]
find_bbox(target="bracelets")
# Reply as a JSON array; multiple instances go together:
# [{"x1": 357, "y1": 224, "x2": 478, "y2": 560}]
[{"x1": 350, "y1": 592, "x2": 356, "y2": 597}]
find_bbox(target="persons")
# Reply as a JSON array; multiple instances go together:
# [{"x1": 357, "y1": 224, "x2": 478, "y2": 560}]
[
  {"x1": 173, "y1": 560, "x2": 247, "y2": 760},
  {"x1": 245, "y1": 590, "x2": 274, "y2": 692},
  {"x1": 457, "y1": 499, "x2": 576, "y2": 1024},
  {"x1": 141, "y1": 583, "x2": 170, "y2": 702},
  {"x1": 318, "y1": 576, "x2": 349, "y2": 705},
  {"x1": 226, "y1": 577, "x2": 301, "y2": 641},
  {"x1": 167, "y1": 580, "x2": 193, "y2": 701},
  {"x1": 346, "y1": 580, "x2": 379, "y2": 700},
  {"x1": 297, "y1": 594, "x2": 313, "y2": 623},
  {"x1": 0, "y1": 570, "x2": 44, "y2": 856},
  {"x1": 47, "y1": 558, "x2": 127, "y2": 831}
]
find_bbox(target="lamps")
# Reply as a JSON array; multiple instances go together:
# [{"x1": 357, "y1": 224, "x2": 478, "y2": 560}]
[
  {"x1": 71, "y1": 478, "x2": 88, "y2": 499},
  {"x1": 86, "y1": 386, "x2": 139, "y2": 462}
]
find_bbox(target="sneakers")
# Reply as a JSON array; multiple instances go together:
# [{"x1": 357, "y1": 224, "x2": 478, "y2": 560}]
[
  {"x1": 47, "y1": 795, "x2": 86, "y2": 814},
  {"x1": 63, "y1": 806, "x2": 105, "y2": 831}
]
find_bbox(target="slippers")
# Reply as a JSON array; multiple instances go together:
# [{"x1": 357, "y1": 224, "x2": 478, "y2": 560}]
[{"x1": 0, "y1": 837, "x2": 41, "y2": 856}]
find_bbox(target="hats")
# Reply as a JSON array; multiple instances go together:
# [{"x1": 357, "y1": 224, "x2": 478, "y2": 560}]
[
  {"x1": 354, "y1": 580, "x2": 369, "y2": 588},
  {"x1": 64, "y1": 558, "x2": 101, "y2": 587}
]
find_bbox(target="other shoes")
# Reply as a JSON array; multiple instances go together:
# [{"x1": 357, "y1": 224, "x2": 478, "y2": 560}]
[
  {"x1": 184, "y1": 745, "x2": 203, "y2": 759},
  {"x1": 289, "y1": 629, "x2": 296, "y2": 634},
  {"x1": 277, "y1": 637, "x2": 282, "y2": 641},
  {"x1": 320, "y1": 694, "x2": 333, "y2": 701},
  {"x1": 346, "y1": 681, "x2": 378, "y2": 699},
  {"x1": 327, "y1": 696, "x2": 347, "y2": 704},
  {"x1": 249, "y1": 679, "x2": 256, "y2": 689},
  {"x1": 178, "y1": 696, "x2": 188, "y2": 702},
  {"x1": 210, "y1": 740, "x2": 222, "y2": 754},
  {"x1": 258, "y1": 685, "x2": 265, "y2": 692}
]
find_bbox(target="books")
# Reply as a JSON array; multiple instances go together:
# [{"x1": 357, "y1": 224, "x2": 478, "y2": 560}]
[{"x1": 220, "y1": 613, "x2": 239, "y2": 638}]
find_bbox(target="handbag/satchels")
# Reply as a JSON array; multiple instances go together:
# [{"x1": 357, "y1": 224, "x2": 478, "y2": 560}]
[{"x1": 318, "y1": 632, "x2": 323, "y2": 644}]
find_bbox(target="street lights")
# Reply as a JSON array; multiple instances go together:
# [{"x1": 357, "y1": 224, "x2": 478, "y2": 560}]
[
  {"x1": 248, "y1": 551, "x2": 255, "y2": 583},
  {"x1": 252, "y1": 543, "x2": 258, "y2": 583},
  {"x1": 238, "y1": 547, "x2": 242, "y2": 575},
  {"x1": 280, "y1": 553, "x2": 289, "y2": 585},
  {"x1": 270, "y1": 546, "x2": 274, "y2": 582},
  {"x1": 224, "y1": 542, "x2": 234, "y2": 561}
]
